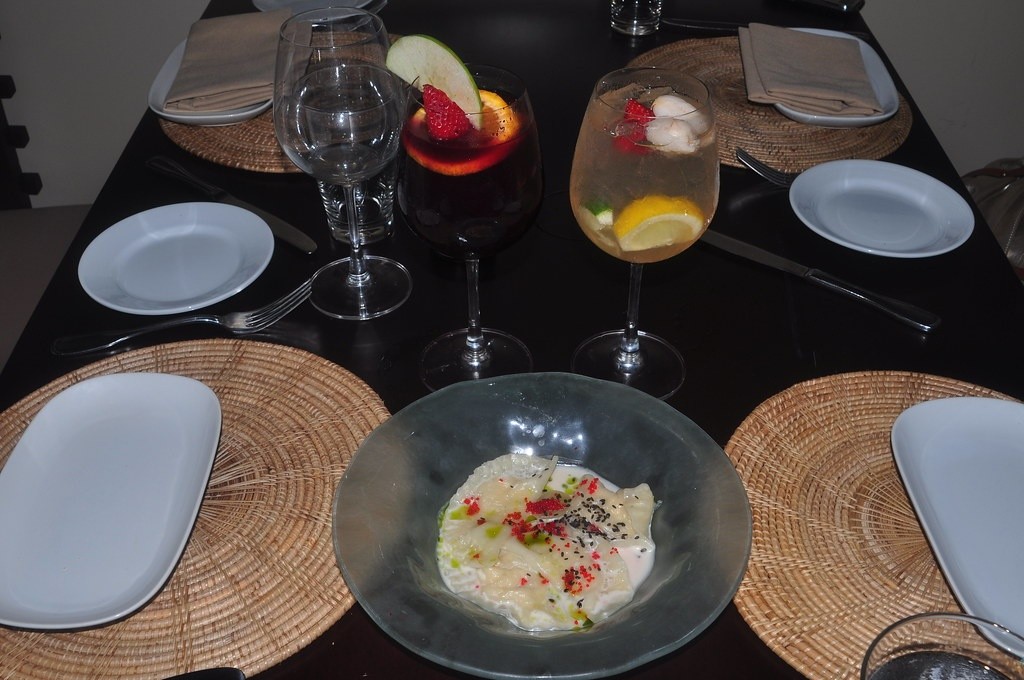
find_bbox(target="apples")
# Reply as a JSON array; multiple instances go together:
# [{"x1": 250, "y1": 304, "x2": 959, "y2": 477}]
[{"x1": 385, "y1": 33, "x2": 483, "y2": 132}]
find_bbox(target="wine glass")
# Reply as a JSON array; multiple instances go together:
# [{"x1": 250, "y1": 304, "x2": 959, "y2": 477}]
[
  {"x1": 569, "y1": 63, "x2": 720, "y2": 404},
  {"x1": 397, "y1": 62, "x2": 546, "y2": 393},
  {"x1": 272, "y1": 6, "x2": 414, "y2": 322}
]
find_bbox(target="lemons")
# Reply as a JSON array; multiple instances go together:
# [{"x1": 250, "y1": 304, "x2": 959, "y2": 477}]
[
  {"x1": 613, "y1": 195, "x2": 704, "y2": 251},
  {"x1": 579, "y1": 200, "x2": 613, "y2": 230}
]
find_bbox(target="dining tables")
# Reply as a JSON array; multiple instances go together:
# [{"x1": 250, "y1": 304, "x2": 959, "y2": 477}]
[{"x1": 0, "y1": 0, "x2": 1024, "y2": 680}]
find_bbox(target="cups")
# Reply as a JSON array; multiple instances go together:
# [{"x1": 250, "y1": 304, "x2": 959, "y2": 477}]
[
  {"x1": 290, "y1": 63, "x2": 397, "y2": 244},
  {"x1": 860, "y1": 611, "x2": 1024, "y2": 680},
  {"x1": 608, "y1": 0, "x2": 661, "y2": 36}
]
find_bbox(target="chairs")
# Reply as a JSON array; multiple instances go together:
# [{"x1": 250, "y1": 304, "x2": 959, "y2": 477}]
[{"x1": 0, "y1": 74, "x2": 93, "y2": 369}]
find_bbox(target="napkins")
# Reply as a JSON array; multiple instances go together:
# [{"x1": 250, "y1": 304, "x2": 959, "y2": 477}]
[
  {"x1": 163, "y1": 7, "x2": 313, "y2": 112},
  {"x1": 738, "y1": 23, "x2": 883, "y2": 115}
]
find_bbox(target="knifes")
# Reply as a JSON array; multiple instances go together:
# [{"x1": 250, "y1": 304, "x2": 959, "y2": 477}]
[
  {"x1": 662, "y1": 18, "x2": 870, "y2": 39},
  {"x1": 698, "y1": 227, "x2": 944, "y2": 335},
  {"x1": 144, "y1": 154, "x2": 320, "y2": 255}
]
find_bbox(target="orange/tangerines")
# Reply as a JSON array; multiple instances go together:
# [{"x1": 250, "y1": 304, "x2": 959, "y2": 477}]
[{"x1": 409, "y1": 86, "x2": 519, "y2": 146}]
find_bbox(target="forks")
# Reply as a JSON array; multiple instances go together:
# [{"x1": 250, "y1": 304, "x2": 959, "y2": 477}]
[
  {"x1": 735, "y1": 146, "x2": 800, "y2": 187},
  {"x1": 49, "y1": 271, "x2": 319, "y2": 358}
]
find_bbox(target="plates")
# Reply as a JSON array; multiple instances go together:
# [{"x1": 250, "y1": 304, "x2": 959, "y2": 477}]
[
  {"x1": 147, "y1": 36, "x2": 275, "y2": 125},
  {"x1": 253, "y1": 0, "x2": 376, "y2": 19},
  {"x1": 785, "y1": 159, "x2": 975, "y2": 258},
  {"x1": 0, "y1": 372, "x2": 222, "y2": 629},
  {"x1": 332, "y1": 369, "x2": 752, "y2": 679},
  {"x1": 892, "y1": 397, "x2": 1024, "y2": 660},
  {"x1": 774, "y1": 26, "x2": 899, "y2": 126},
  {"x1": 77, "y1": 200, "x2": 275, "y2": 318}
]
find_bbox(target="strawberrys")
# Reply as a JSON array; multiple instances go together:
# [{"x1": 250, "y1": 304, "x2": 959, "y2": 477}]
[
  {"x1": 613, "y1": 99, "x2": 658, "y2": 156},
  {"x1": 422, "y1": 84, "x2": 472, "y2": 141}
]
